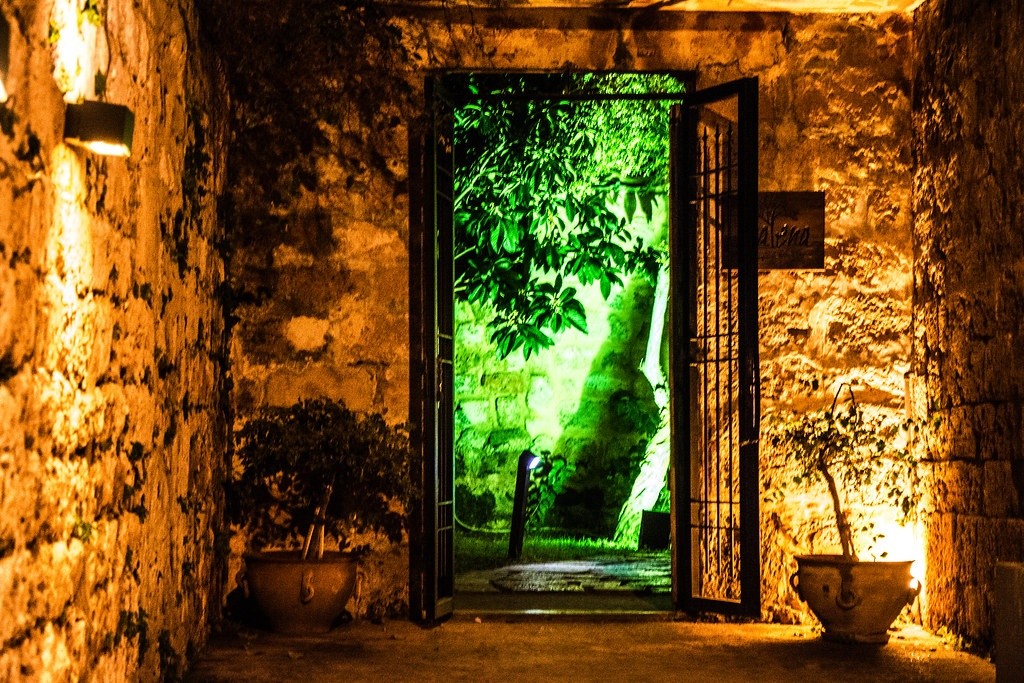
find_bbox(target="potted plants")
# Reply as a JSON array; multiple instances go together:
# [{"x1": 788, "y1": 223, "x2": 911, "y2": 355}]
[
  {"x1": 217, "y1": 394, "x2": 422, "y2": 639},
  {"x1": 768, "y1": 383, "x2": 928, "y2": 646}
]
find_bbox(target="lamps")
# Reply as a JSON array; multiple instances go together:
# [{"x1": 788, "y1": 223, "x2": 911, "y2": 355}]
[{"x1": 63, "y1": 101, "x2": 135, "y2": 158}]
